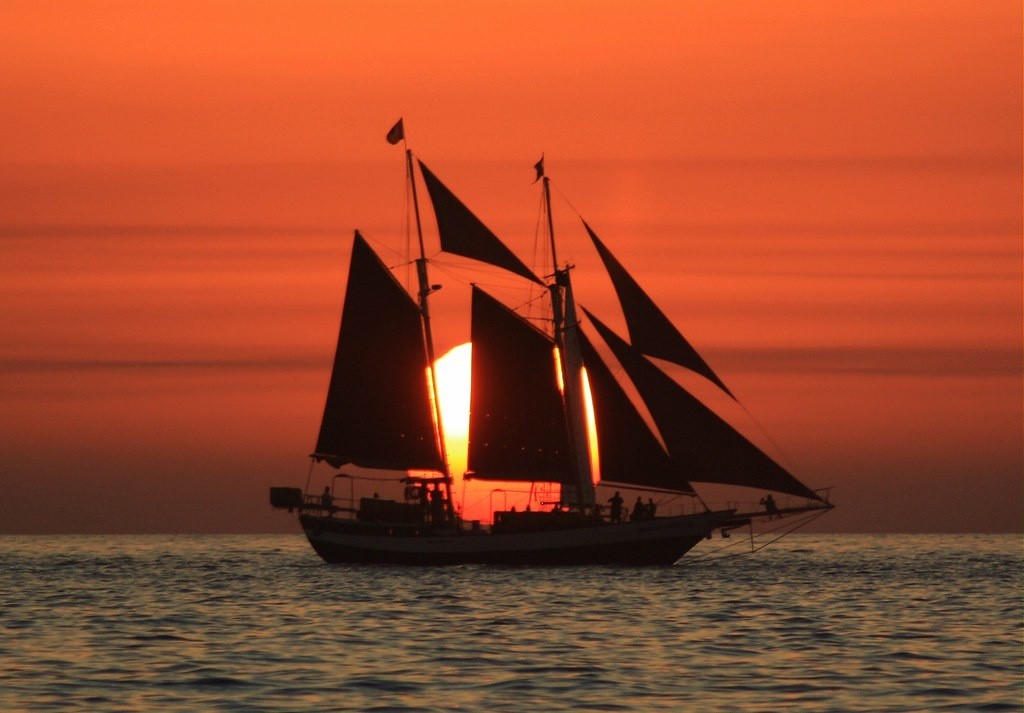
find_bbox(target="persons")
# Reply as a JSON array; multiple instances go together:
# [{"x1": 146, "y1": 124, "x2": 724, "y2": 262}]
[
  {"x1": 760, "y1": 495, "x2": 782, "y2": 519},
  {"x1": 320, "y1": 485, "x2": 338, "y2": 518},
  {"x1": 608, "y1": 492, "x2": 656, "y2": 523}
]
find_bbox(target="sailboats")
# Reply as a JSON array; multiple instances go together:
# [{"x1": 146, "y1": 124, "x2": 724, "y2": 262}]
[{"x1": 267, "y1": 118, "x2": 836, "y2": 573}]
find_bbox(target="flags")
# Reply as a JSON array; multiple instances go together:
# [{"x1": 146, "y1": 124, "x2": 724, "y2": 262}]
[
  {"x1": 533, "y1": 157, "x2": 544, "y2": 185},
  {"x1": 386, "y1": 117, "x2": 404, "y2": 145}
]
